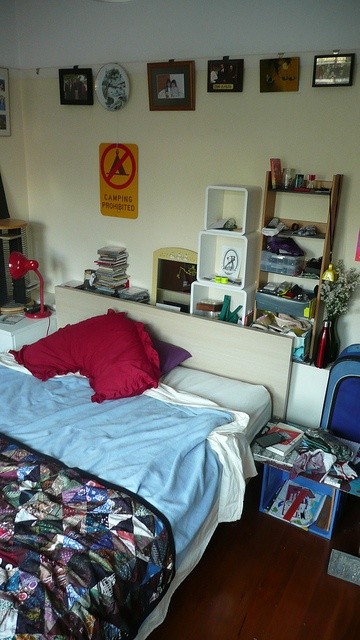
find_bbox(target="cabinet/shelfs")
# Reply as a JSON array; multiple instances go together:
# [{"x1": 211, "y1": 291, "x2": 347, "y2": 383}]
[{"x1": 252, "y1": 157, "x2": 344, "y2": 366}]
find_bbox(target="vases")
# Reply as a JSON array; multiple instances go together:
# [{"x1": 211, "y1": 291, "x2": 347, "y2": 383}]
[{"x1": 326, "y1": 315, "x2": 340, "y2": 363}]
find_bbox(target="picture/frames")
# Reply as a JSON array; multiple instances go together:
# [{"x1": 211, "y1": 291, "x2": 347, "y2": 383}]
[
  {"x1": 311, "y1": 52, "x2": 355, "y2": 87},
  {"x1": 152, "y1": 247, "x2": 197, "y2": 308},
  {"x1": 146, "y1": 61, "x2": 195, "y2": 112},
  {"x1": 259, "y1": 56, "x2": 301, "y2": 92},
  {"x1": 207, "y1": 58, "x2": 245, "y2": 93},
  {"x1": 95, "y1": 63, "x2": 131, "y2": 112},
  {"x1": 0, "y1": 68, "x2": 12, "y2": 138},
  {"x1": 58, "y1": 68, "x2": 93, "y2": 105}
]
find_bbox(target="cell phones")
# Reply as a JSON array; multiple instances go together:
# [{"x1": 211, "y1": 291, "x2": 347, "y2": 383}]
[
  {"x1": 256, "y1": 432, "x2": 286, "y2": 448},
  {"x1": 23, "y1": 301, "x2": 49, "y2": 317}
]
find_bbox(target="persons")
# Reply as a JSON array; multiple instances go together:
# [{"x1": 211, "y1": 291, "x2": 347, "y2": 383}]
[
  {"x1": 165, "y1": 80, "x2": 178, "y2": 98},
  {"x1": 210, "y1": 63, "x2": 237, "y2": 85}
]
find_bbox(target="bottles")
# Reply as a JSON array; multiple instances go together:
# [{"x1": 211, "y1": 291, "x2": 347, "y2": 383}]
[
  {"x1": 306, "y1": 171, "x2": 316, "y2": 190},
  {"x1": 314, "y1": 319, "x2": 331, "y2": 369},
  {"x1": 281, "y1": 168, "x2": 295, "y2": 189},
  {"x1": 294, "y1": 174, "x2": 305, "y2": 188}
]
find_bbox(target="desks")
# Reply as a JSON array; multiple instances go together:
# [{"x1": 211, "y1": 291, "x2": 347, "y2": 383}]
[{"x1": 250, "y1": 419, "x2": 360, "y2": 541}]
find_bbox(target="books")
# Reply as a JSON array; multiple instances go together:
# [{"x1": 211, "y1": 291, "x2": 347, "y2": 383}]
[
  {"x1": 261, "y1": 423, "x2": 304, "y2": 459},
  {"x1": 95, "y1": 247, "x2": 131, "y2": 293},
  {"x1": 268, "y1": 479, "x2": 325, "y2": 530}
]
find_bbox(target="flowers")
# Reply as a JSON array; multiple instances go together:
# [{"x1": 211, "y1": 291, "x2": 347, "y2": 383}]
[{"x1": 320, "y1": 258, "x2": 360, "y2": 316}]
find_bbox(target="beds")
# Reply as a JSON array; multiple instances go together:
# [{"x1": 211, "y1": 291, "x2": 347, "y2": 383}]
[{"x1": 0, "y1": 284, "x2": 294, "y2": 620}]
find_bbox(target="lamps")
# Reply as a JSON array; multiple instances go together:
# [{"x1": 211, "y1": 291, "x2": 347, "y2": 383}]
[
  {"x1": 9, "y1": 252, "x2": 52, "y2": 319},
  {"x1": 322, "y1": 263, "x2": 337, "y2": 282}
]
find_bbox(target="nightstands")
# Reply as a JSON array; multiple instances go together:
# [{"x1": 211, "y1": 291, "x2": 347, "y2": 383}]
[{"x1": 0, "y1": 286, "x2": 57, "y2": 353}]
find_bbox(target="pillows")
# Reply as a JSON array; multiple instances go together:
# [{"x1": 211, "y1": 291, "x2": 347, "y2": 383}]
[
  {"x1": 150, "y1": 337, "x2": 193, "y2": 378},
  {"x1": 8, "y1": 308, "x2": 161, "y2": 404}
]
